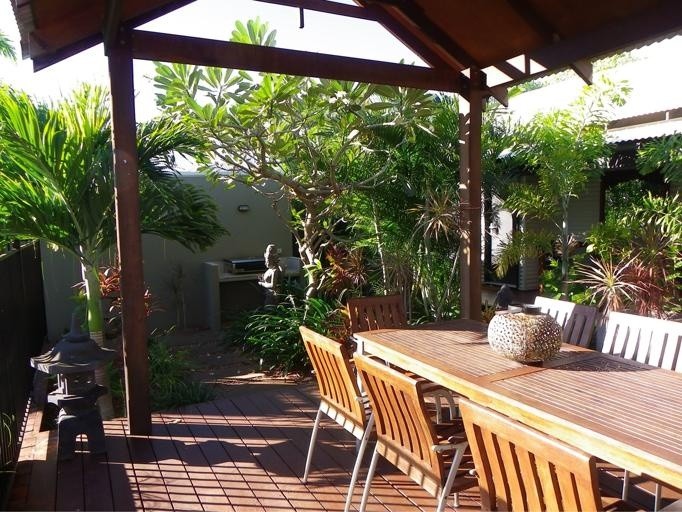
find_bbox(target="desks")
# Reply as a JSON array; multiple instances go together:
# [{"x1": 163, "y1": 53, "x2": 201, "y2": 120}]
[{"x1": 349, "y1": 317, "x2": 682, "y2": 512}]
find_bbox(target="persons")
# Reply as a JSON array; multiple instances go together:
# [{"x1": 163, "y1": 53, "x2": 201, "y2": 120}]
[{"x1": 259, "y1": 243, "x2": 282, "y2": 306}]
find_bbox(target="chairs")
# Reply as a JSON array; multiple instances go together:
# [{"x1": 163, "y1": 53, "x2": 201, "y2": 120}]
[
  {"x1": 457, "y1": 396, "x2": 628, "y2": 512},
  {"x1": 352, "y1": 350, "x2": 470, "y2": 512},
  {"x1": 346, "y1": 293, "x2": 412, "y2": 371},
  {"x1": 600, "y1": 310, "x2": 682, "y2": 374},
  {"x1": 534, "y1": 295, "x2": 598, "y2": 349},
  {"x1": 299, "y1": 324, "x2": 379, "y2": 512}
]
